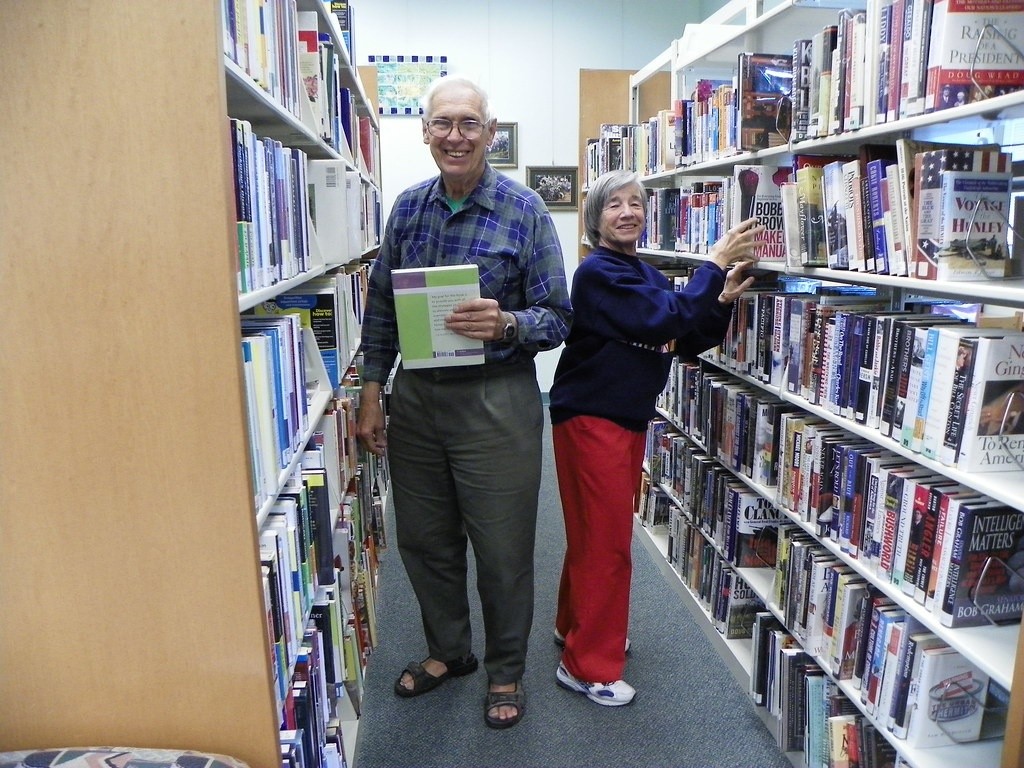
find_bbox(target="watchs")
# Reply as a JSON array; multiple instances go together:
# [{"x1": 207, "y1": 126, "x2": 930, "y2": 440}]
[{"x1": 499, "y1": 313, "x2": 515, "y2": 342}]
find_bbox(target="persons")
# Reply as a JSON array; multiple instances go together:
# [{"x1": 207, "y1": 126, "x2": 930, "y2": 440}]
[
  {"x1": 954, "y1": 92, "x2": 965, "y2": 107},
  {"x1": 941, "y1": 85, "x2": 953, "y2": 109},
  {"x1": 357, "y1": 76, "x2": 571, "y2": 730},
  {"x1": 549, "y1": 170, "x2": 767, "y2": 706},
  {"x1": 806, "y1": 440, "x2": 812, "y2": 454},
  {"x1": 910, "y1": 508, "x2": 925, "y2": 544},
  {"x1": 956, "y1": 347, "x2": 965, "y2": 371}
]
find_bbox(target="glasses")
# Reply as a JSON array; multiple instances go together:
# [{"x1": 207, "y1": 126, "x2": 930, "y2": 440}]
[{"x1": 425, "y1": 118, "x2": 491, "y2": 140}]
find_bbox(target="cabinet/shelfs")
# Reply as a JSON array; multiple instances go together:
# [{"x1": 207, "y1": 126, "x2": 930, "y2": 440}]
[
  {"x1": 0, "y1": 1, "x2": 392, "y2": 768},
  {"x1": 581, "y1": 0, "x2": 1023, "y2": 768}
]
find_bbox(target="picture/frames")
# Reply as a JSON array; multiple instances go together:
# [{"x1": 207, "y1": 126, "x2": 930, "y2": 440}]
[
  {"x1": 368, "y1": 56, "x2": 448, "y2": 116},
  {"x1": 485, "y1": 123, "x2": 518, "y2": 168},
  {"x1": 526, "y1": 165, "x2": 580, "y2": 211}
]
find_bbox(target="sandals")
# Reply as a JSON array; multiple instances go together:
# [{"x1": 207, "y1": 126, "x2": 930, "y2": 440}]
[
  {"x1": 486, "y1": 677, "x2": 526, "y2": 729},
  {"x1": 395, "y1": 652, "x2": 480, "y2": 696}
]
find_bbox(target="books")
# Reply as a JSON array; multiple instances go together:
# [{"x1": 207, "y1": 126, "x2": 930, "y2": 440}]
[
  {"x1": 390, "y1": 264, "x2": 485, "y2": 369},
  {"x1": 584, "y1": 0, "x2": 1024, "y2": 768},
  {"x1": 221, "y1": 0, "x2": 387, "y2": 768}
]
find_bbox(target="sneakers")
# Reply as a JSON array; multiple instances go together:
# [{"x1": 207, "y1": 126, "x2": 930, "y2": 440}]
[
  {"x1": 556, "y1": 660, "x2": 636, "y2": 706},
  {"x1": 554, "y1": 628, "x2": 632, "y2": 653}
]
